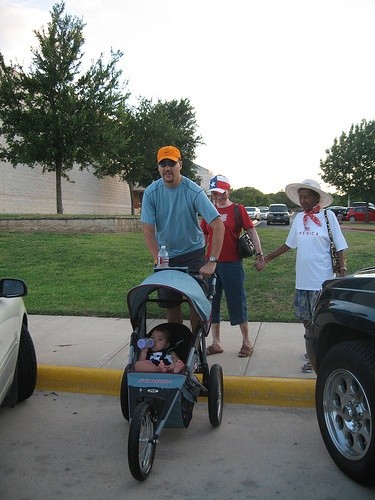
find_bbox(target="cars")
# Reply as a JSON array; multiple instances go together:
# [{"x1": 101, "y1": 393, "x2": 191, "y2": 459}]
[
  {"x1": 245, "y1": 205, "x2": 269, "y2": 222},
  {"x1": 0, "y1": 277, "x2": 38, "y2": 410},
  {"x1": 326, "y1": 201, "x2": 375, "y2": 221}
]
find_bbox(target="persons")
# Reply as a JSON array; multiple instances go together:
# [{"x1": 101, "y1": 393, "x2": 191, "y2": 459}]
[
  {"x1": 134, "y1": 327, "x2": 185, "y2": 374},
  {"x1": 141, "y1": 146, "x2": 225, "y2": 373},
  {"x1": 254, "y1": 179, "x2": 348, "y2": 373},
  {"x1": 288, "y1": 208, "x2": 295, "y2": 228},
  {"x1": 337, "y1": 211, "x2": 344, "y2": 231},
  {"x1": 200, "y1": 174, "x2": 266, "y2": 358}
]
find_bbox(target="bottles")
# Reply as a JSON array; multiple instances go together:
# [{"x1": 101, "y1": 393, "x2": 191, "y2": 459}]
[
  {"x1": 137, "y1": 338, "x2": 157, "y2": 348},
  {"x1": 157, "y1": 246, "x2": 170, "y2": 268}
]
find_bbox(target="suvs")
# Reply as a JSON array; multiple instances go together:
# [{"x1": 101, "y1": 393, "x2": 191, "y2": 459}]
[
  {"x1": 265, "y1": 203, "x2": 291, "y2": 225},
  {"x1": 303, "y1": 265, "x2": 375, "y2": 489}
]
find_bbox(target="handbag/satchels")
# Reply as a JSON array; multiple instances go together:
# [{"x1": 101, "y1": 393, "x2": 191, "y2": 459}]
[
  {"x1": 328, "y1": 242, "x2": 348, "y2": 273},
  {"x1": 238, "y1": 232, "x2": 255, "y2": 257}
]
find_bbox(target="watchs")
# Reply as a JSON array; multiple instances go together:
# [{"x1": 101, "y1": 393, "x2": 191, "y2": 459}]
[
  {"x1": 255, "y1": 251, "x2": 263, "y2": 256},
  {"x1": 340, "y1": 267, "x2": 347, "y2": 271},
  {"x1": 209, "y1": 256, "x2": 218, "y2": 262}
]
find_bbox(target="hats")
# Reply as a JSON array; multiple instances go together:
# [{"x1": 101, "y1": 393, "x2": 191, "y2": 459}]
[
  {"x1": 208, "y1": 174, "x2": 231, "y2": 194},
  {"x1": 157, "y1": 145, "x2": 180, "y2": 162},
  {"x1": 284, "y1": 179, "x2": 332, "y2": 208}
]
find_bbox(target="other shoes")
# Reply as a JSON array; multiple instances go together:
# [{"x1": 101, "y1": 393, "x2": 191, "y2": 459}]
[
  {"x1": 205, "y1": 346, "x2": 224, "y2": 356},
  {"x1": 302, "y1": 361, "x2": 315, "y2": 373},
  {"x1": 303, "y1": 353, "x2": 309, "y2": 360},
  {"x1": 238, "y1": 345, "x2": 253, "y2": 357}
]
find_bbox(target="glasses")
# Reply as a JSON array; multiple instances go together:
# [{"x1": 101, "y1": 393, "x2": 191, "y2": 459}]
[
  {"x1": 212, "y1": 192, "x2": 226, "y2": 196},
  {"x1": 159, "y1": 161, "x2": 181, "y2": 167}
]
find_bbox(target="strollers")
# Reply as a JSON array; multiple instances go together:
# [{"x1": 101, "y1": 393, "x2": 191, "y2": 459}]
[{"x1": 119, "y1": 266, "x2": 226, "y2": 482}]
